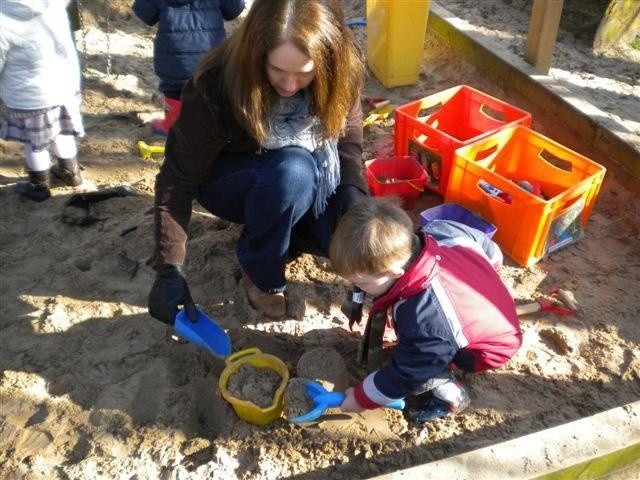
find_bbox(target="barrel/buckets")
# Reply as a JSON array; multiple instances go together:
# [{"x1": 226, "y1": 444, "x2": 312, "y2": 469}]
[
  {"x1": 214, "y1": 347, "x2": 292, "y2": 427},
  {"x1": 419, "y1": 203, "x2": 498, "y2": 240},
  {"x1": 365, "y1": 155, "x2": 427, "y2": 211}
]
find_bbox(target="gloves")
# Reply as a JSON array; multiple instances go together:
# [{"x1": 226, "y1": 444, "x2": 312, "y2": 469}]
[{"x1": 148, "y1": 265, "x2": 200, "y2": 325}]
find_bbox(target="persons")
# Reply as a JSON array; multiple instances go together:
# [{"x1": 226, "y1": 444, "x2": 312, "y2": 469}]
[
  {"x1": 0, "y1": 0, "x2": 85, "y2": 200},
  {"x1": 329, "y1": 196, "x2": 522, "y2": 421},
  {"x1": 132, "y1": 0, "x2": 370, "y2": 326},
  {"x1": 133, "y1": 2, "x2": 244, "y2": 136}
]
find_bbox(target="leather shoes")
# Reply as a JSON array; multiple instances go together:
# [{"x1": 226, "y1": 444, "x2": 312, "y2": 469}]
[{"x1": 239, "y1": 264, "x2": 287, "y2": 319}]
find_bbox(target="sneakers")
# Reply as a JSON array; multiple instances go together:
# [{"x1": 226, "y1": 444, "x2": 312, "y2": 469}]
[{"x1": 408, "y1": 378, "x2": 470, "y2": 421}]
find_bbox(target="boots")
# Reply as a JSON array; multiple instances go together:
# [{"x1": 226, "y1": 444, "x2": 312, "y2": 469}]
[
  {"x1": 151, "y1": 98, "x2": 183, "y2": 137},
  {"x1": 17, "y1": 170, "x2": 52, "y2": 201},
  {"x1": 52, "y1": 156, "x2": 82, "y2": 186}
]
